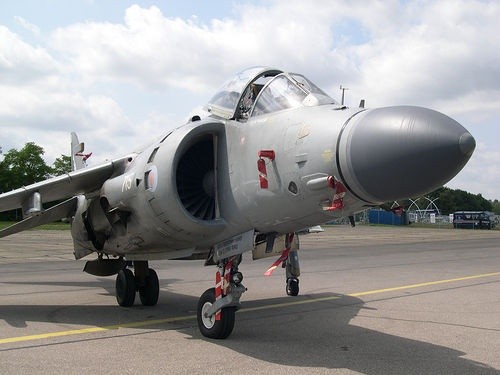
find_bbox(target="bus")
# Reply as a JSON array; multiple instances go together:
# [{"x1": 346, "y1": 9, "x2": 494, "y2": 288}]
[{"x1": 452, "y1": 210, "x2": 498, "y2": 230}]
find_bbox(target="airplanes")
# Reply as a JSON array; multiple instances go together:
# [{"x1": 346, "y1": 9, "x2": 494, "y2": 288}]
[{"x1": 1, "y1": 63, "x2": 477, "y2": 340}]
